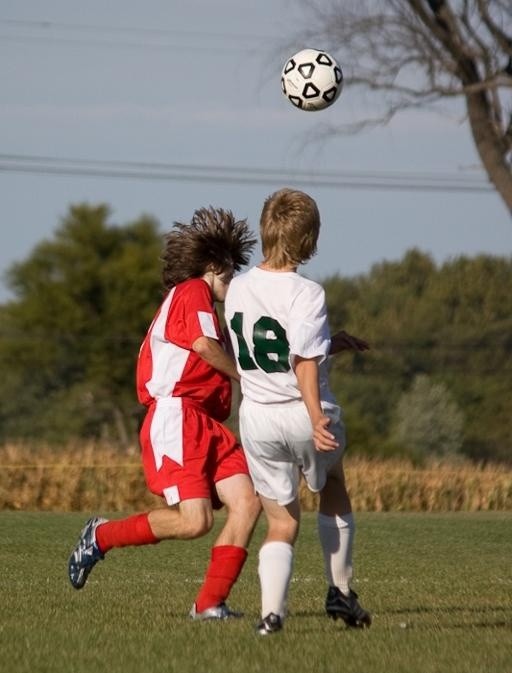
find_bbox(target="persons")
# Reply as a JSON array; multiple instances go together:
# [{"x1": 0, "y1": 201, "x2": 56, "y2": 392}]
[
  {"x1": 67, "y1": 203, "x2": 264, "y2": 627},
  {"x1": 223, "y1": 186, "x2": 373, "y2": 636}
]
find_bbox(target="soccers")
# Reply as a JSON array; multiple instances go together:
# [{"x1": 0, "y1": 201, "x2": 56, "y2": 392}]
[{"x1": 281, "y1": 48, "x2": 343, "y2": 111}]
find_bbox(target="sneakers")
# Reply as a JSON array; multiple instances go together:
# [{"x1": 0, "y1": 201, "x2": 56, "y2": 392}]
[
  {"x1": 254, "y1": 611, "x2": 286, "y2": 637},
  {"x1": 188, "y1": 597, "x2": 246, "y2": 624},
  {"x1": 325, "y1": 582, "x2": 374, "y2": 630},
  {"x1": 65, "y1": 515, "x2": 114, "y2": 590}
]
